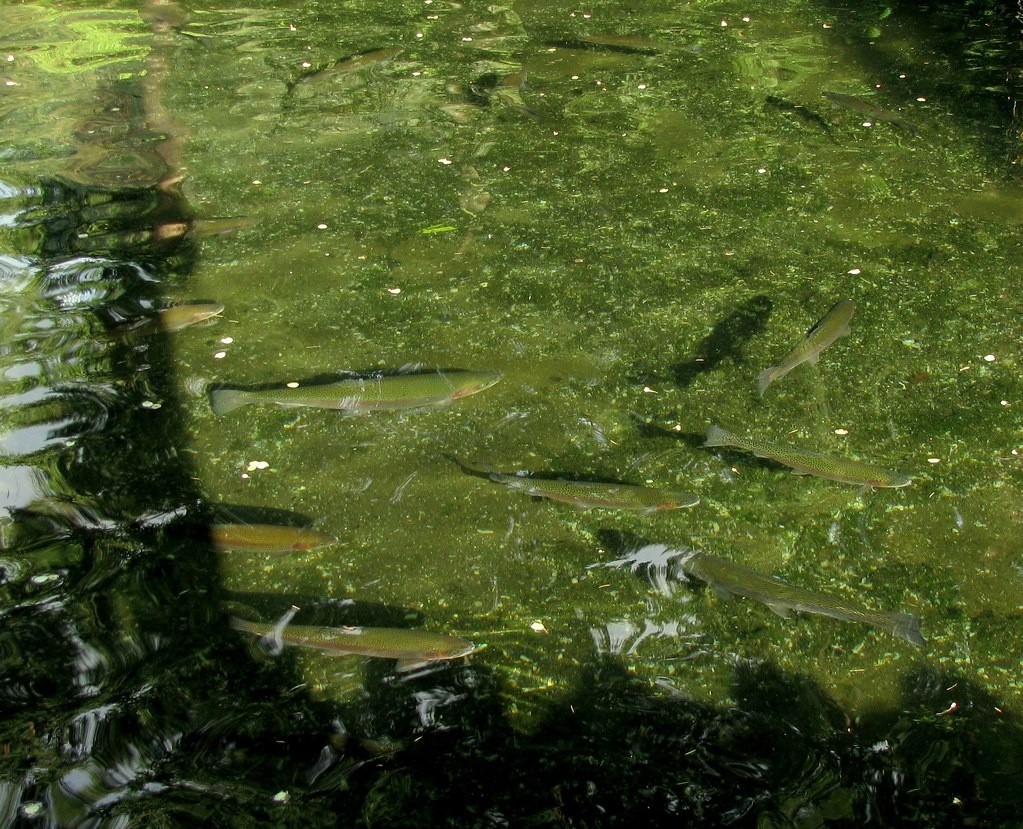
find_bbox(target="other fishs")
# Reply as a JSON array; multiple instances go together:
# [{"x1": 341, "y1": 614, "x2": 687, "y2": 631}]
[{"x1": 89, "y1": 28, "x2": 930, "y2": 676}]
